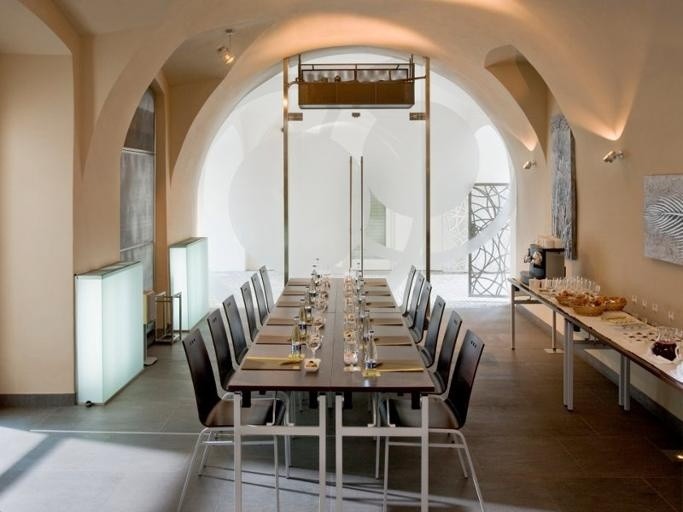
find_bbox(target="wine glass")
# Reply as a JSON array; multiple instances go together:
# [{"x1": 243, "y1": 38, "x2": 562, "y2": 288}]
[
  {"x1": 307, "y1": 271, "x2": 330, "y2": 357},
  {"x1": 343, "y1": 274, "x2": 361, "y2": 372}
]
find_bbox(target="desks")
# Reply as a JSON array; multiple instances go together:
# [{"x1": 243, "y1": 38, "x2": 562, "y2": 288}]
[{"x1": 507, "y1": 278, "x2": 683, "y2": 413}]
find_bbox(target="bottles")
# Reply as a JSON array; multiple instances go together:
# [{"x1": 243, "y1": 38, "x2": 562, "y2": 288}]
[
  {"x1": 354, "y1": 263, "x2": 378, "y2": 370},
  {"x1": 290, "y1": 258, "x2": 319, "y2": 359},
  {"x1": 650, "y1": 327, "x2": 678, "y2": 360}
]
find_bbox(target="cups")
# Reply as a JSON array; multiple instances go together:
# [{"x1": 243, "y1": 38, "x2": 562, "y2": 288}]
[{"x1": 527, "y1": 277, "x2": 601, "y2": 297}]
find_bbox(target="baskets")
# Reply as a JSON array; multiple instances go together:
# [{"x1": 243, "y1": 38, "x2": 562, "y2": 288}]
[{"x1": 555, "y1": 293, "x2": 627, "y2": 316}]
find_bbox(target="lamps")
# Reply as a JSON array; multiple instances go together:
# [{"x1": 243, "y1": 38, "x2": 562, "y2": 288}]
[
  {"x1": 603, "y1": 150, "x2": 623, "y2": 163},
  {"x1": 523, "y1": 160, "x2": 535, "y2": 169},
  {"x1": 218, "y1": 29, "x2": 235, "y2": 64}
]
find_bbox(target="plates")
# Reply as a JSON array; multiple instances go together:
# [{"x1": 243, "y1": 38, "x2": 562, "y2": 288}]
[{"x1": 534, "y1": 233, "x2": 563, "y2": 249}]
